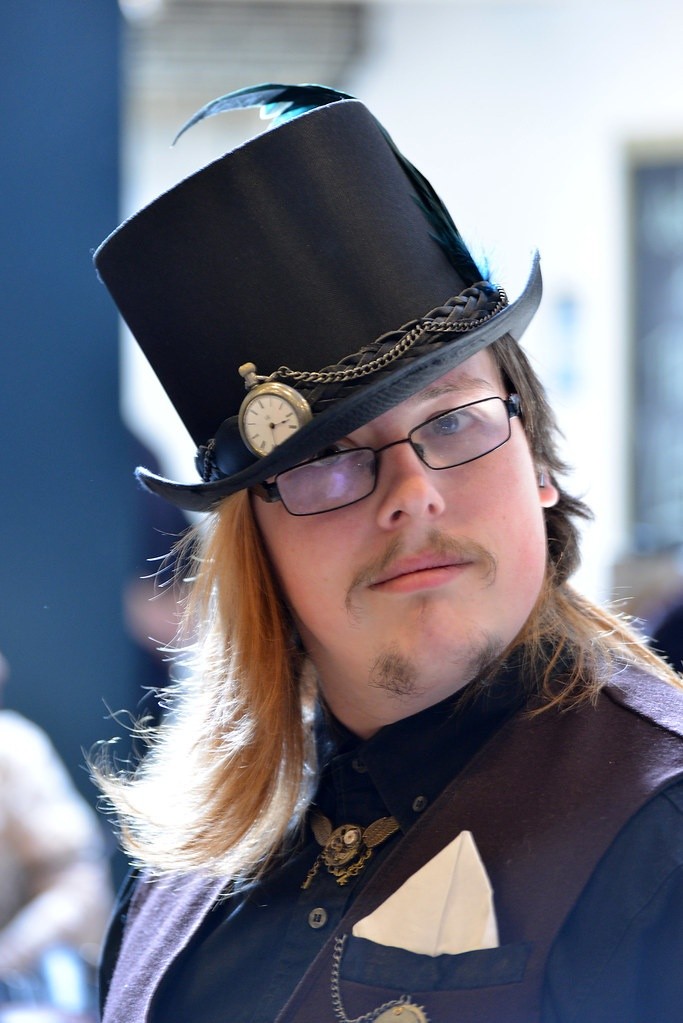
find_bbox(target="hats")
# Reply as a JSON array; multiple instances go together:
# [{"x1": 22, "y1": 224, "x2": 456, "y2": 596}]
[{"x1": 87, "y1": 84, "x2": 554, "y2": 512}]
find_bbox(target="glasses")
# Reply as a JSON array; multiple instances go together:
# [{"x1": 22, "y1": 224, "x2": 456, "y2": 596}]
[{"x1": 251, "y1": 395, "x2": 526, "y2": 517}]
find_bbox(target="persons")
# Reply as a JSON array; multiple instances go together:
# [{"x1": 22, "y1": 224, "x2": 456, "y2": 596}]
[
  {"x1": 2, "y1": 707, "x2": 116, "y2": 979},
  {"x1": 98, "y1": 98, "x2": 683, "y2": 1023}
]
